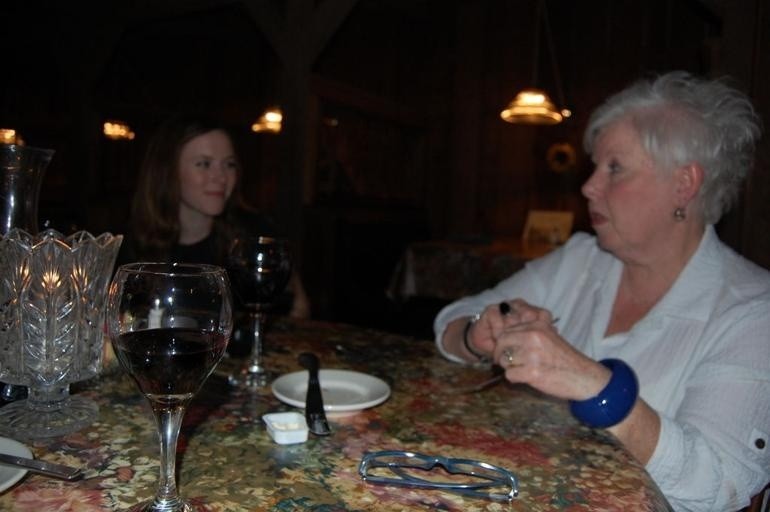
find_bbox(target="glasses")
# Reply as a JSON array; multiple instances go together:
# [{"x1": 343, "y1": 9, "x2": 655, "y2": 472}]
[{"x1": 350, "y1": 447, "x2": 522, "y2": 505}]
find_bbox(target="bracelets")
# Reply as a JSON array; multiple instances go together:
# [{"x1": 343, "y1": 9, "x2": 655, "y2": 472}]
[
  {"x1": 569, "y1": 359, "x2": 639, "y2": 431},
  {"x1": 462, "y1": 314, "x2": 489, "y2": 363}
]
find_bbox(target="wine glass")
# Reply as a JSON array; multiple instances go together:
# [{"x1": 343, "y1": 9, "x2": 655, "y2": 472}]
[
  {"x1": 105, "y1": 260, "x2": 234, "y2": 512},
  {"x1": 0, "y1": 229, "x2": 126, "y2": 439},
  {"x1": 227, "y1": 235, "x2": 292, "y2": 391}
]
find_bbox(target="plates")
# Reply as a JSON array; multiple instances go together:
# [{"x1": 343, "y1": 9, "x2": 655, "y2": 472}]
[
  {"x1": 0, "y1": 436, "x2": 34, "y2": 493},
  {"x1": 270, "y1": 367, "x2": 391, "y2": 412}
]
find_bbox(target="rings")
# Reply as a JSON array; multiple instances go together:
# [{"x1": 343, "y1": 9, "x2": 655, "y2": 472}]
[
  {"x1": 499, "y1": 301, "x2": 514, "y2": 317},
  {"x1": 503, "y1": 347, "x2": 513, "y2": 366}
]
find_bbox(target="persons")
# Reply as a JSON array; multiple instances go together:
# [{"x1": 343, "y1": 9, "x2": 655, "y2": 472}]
[
  {"x1": 432, "y1": 68, "x2": 769, "y2": 511},
  {"x1": 117, "y1": 114, "x2": 312, "y2": 329}
]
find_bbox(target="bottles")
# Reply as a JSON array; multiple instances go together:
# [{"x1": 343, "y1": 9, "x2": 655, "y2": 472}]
[{"x1": 0, "y1": 142, "x2": 56, "y2": 236}]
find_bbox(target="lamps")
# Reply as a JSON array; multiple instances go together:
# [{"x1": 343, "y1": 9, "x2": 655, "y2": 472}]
[{"x1": 500, "y1": 0, "x2": 564, "y2": 128}]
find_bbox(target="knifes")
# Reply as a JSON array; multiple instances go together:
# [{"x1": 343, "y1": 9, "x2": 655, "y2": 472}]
[{"x1": 298, "y1": 352, "x2": 332, "y2": 436}]
